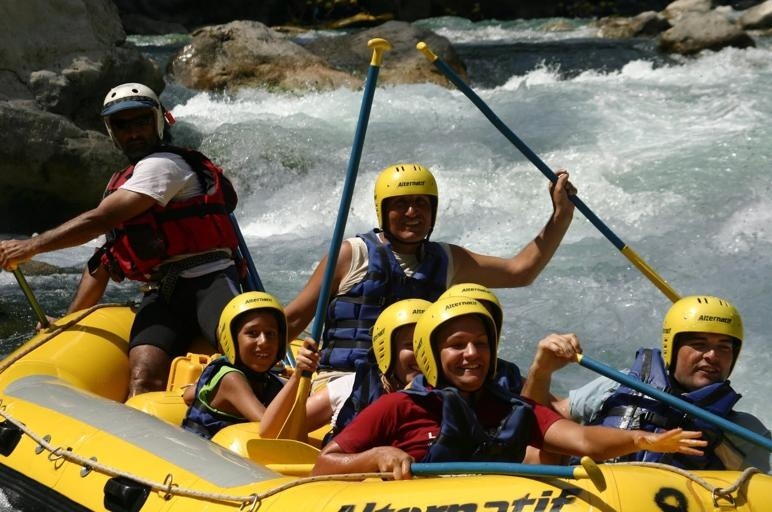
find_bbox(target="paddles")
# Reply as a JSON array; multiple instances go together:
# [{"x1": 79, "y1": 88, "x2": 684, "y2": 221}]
[
  {"x1": 247, "y1": 437, "x2": 607, "y2": 492},
  {"x1": 276, "y1": 38, "x2": 393, "y2": 439},
  {"x1": 417, "y1": 40, "x2": 682, "y2": 305}
]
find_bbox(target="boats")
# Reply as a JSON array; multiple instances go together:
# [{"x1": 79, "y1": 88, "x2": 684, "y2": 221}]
[{"x1": 0, "y1": 296, "x2": 772, "y2": 511}]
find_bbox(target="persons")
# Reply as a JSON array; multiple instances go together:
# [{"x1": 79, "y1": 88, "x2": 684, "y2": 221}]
[
  {"x1": 285, "y1": 161, "x2": 577, "y2": 386},
  {"x1": 182, "y1": 290, "x2": 294, "y2": 439},
  {"x1": 439, "y1": 282, "x2": 528, "y2": 399},
  {"x1": 285, "y1": 0, "x2": 364, "y2": 27},
  {"x1": 310, "y1": 293, "x2": 708, "y2": 483},
  {"x1": 0, "y1": 81, "x2": 242, "y2": 399},
  {"x1": 518, "y1": 296, "x2": 772, "y2": 480},
  {"x1": 259, "y1": 299, "x2": 433, "y2": 442}
]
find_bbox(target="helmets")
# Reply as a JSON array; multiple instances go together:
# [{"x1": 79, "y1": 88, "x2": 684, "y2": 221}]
[
  {"x1": 663, "y1": 296, "x2": 742, "y2": 378},
  {"x1": 217, "y1": 291, "x2": 287, "y2": 366},
  {"x1": 374, "y1": 164, "x2": 438, "y2": 228},
  {"x1": 372, "y1": 283, "x2": 503, "y2": 388},
  {"x1": 101, "y1": 82, "x2": 164, "y2": 153}
]
